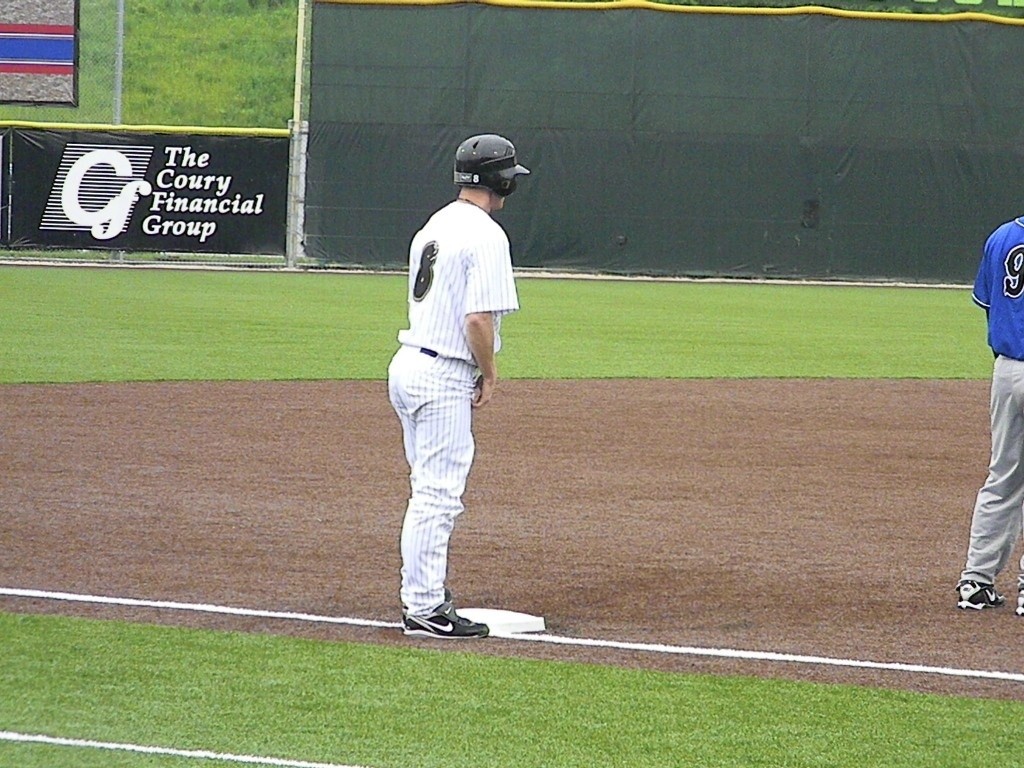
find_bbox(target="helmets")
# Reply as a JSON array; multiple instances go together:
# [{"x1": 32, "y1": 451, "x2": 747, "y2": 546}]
[{"x1": 453, "y1": 134, "x2": 530, "y2": 198}]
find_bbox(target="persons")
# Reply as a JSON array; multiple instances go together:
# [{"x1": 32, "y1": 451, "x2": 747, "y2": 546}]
[
  {"x1": 956, "y1": 215, "x2": 1024, "y2": 619},
  {"x1": 388, "y1": 134, "x2": 530, "y2": 641}
]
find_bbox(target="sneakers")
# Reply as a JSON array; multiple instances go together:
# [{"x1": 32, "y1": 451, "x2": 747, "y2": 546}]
[
  {"x1": 403, "y1": 586, "x2": 453, "y2": 617},
  {"x1": 404, "y1": 604, "x2": 490, "y2": 639},
  {"x1": 1012, "y1": 589, "x2": 1024, "y2": 616},
  {"x1": 955, "y1": 582, "x2": 1003, "y2": 610}
]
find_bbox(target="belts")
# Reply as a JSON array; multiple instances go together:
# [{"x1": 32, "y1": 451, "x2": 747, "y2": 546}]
[{"x1": 420, "y1": 348, "x2": 438, "y2": 358}]
